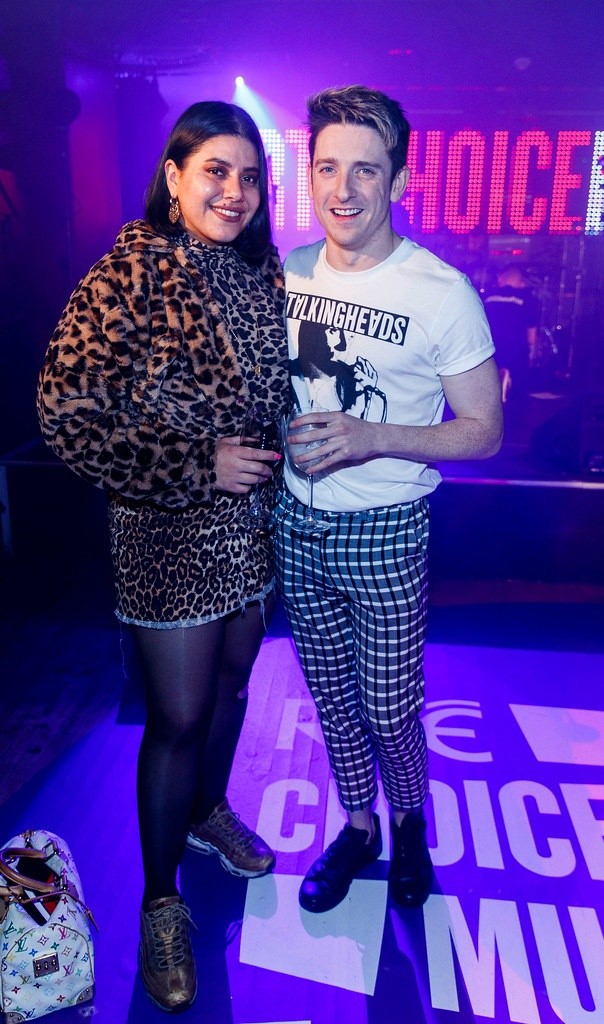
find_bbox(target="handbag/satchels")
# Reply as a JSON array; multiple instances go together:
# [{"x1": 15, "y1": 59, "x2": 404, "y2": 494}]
[{"x1": 0, "y1": 830, "x2": 100, "y2": 1024}]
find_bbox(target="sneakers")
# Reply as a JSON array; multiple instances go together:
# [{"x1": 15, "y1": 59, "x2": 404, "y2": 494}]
[
  {"x1": 298, "y1": 812, "x2": 383, "y2": 913},
  {"x1": 186, "y1": 796, "x2": 277, "y2": 879},
  {"x1": 387, "y1": 804, "x2": 433, "y2": 908},
  {"x1": 140, "y1": 893, "x2": 197, "y2": 1013}
]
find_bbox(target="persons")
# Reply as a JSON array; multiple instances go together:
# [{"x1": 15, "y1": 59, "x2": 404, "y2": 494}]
[
  {"x1": 482, "y1": 261, "x2": 540, "y2": 446},
  {"x1": 269, "y1": 85, "x2": 504, "y2": 913},
  {"x1": 37, "y1": 100, "x2": 293, "y2": 1008}
]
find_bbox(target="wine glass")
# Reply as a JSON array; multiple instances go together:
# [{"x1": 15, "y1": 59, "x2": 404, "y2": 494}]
[
  {"x1": 239, "y1": 406, "x2": 284, "y2": 527},
  {"x1": 286, "y1": 407, "x2": 332, "y2": 533}
]
